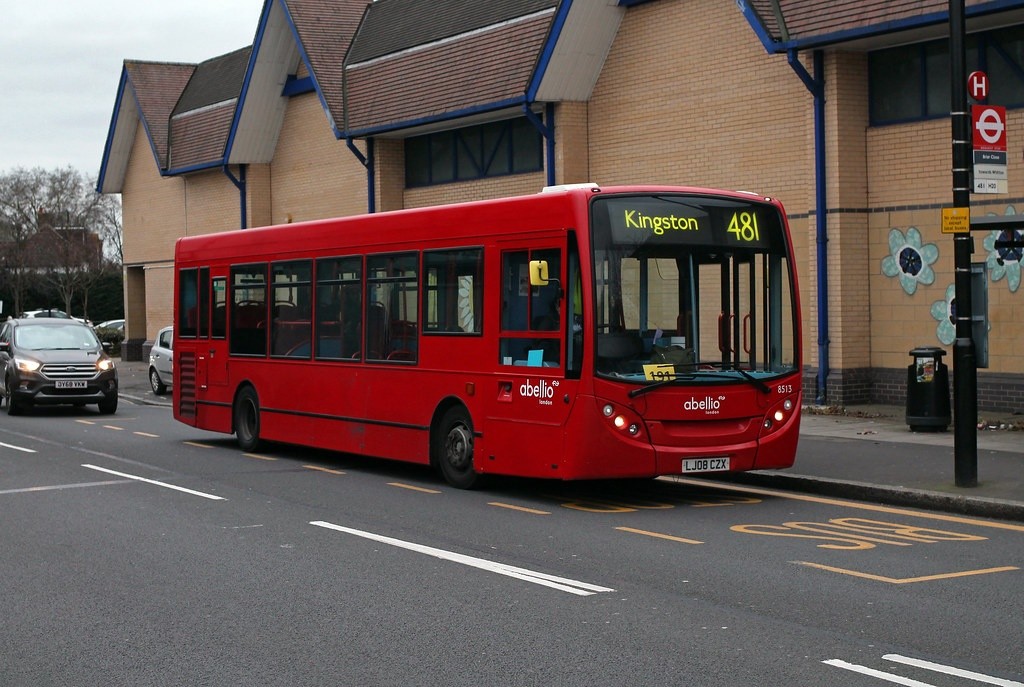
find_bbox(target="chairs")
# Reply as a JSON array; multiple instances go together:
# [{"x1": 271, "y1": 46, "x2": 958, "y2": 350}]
[{"x1": 208, "y1": 297, "x2": 560, "y2": 360}]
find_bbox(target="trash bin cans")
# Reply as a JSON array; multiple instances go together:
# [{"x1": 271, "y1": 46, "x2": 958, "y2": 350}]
[{"x1": 908, "y1": 347, "x2": 951, "y2": 431}]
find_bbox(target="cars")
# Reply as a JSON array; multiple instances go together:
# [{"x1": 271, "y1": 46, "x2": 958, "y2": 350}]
[
  {"x1": 93, "y1": 318, "x2": 126, "y2": 346},
  {"x1": 148, "y1": 326, "x2": 174, "y2": 396}
]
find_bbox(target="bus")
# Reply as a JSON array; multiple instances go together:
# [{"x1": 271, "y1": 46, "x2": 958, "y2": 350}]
[{"x1": 172, "y1": 184, "x2": 803, "y2": 492}]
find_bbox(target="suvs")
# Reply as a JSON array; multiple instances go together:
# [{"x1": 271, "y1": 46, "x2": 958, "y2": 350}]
[
  {"x1": 18, "y1": 307, "x2": 94, "y2": 329},
  {"x1": 0, "y1": 318, "x2": 118, "y2": 417}
]
